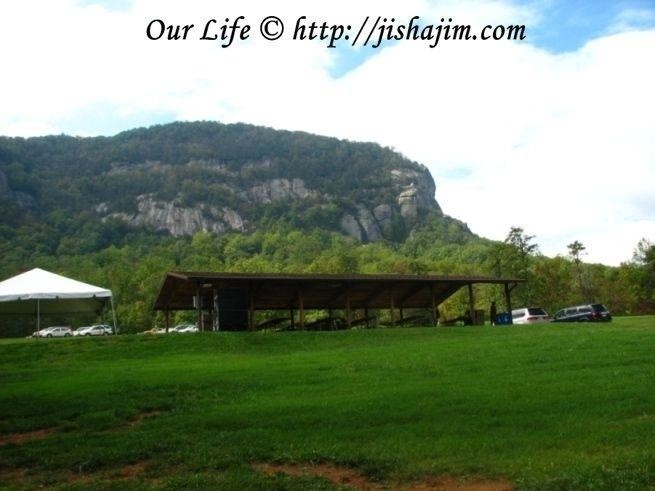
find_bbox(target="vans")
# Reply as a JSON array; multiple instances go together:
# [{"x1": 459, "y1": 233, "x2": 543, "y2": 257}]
[
  {"x1": 490, "y1": 306, "x2": 551, "y2": 326},
  {"x1": 550, "y1": 302, "x2": 613, "y2": 323}
]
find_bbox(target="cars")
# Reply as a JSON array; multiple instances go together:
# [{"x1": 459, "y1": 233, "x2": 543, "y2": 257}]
[{"x1": 142, "y1": 324, "x2": 199, "y2": 335}]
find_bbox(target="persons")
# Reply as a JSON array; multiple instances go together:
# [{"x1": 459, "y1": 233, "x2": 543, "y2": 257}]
[{"x1": 490, "y1": 301, "x2": 497, "y2": 326}]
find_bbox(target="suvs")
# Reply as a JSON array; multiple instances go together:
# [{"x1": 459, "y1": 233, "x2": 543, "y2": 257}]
[{"x1": 29, "y1": 323, "x2": 113, "y2": 339}]
